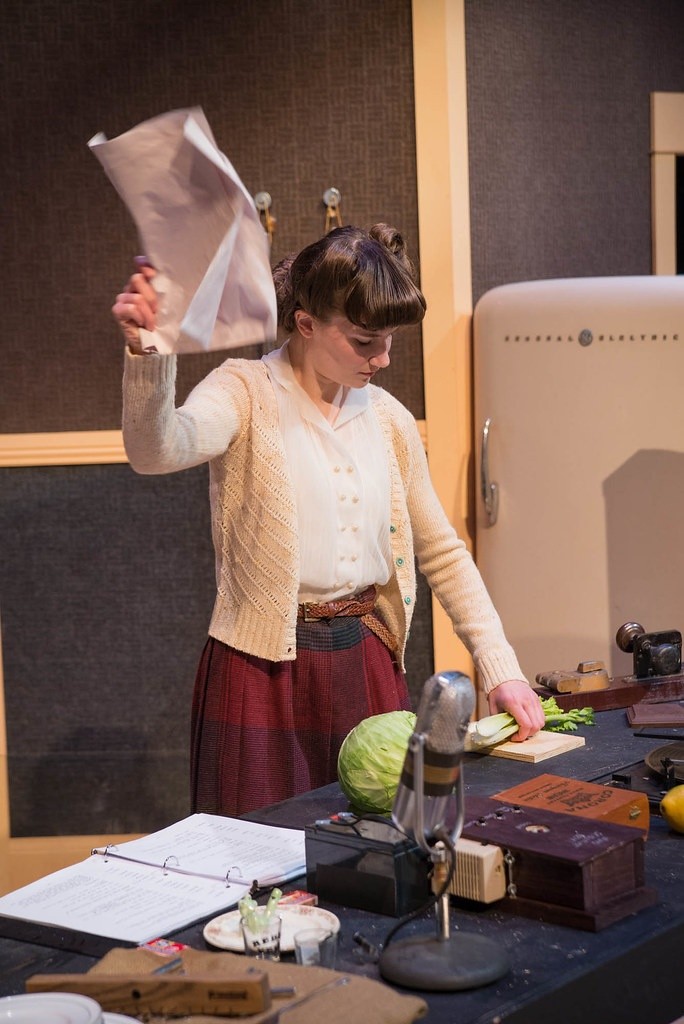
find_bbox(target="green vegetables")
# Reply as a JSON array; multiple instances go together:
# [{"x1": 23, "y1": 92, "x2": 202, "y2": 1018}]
[{"x1": 468, "y1": 696, "x2": 596, "y2": 751}]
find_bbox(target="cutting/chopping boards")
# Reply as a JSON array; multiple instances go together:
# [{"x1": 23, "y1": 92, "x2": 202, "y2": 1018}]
[{"x1": 476, "y1": 730, "x2": 585, "y2": 763}]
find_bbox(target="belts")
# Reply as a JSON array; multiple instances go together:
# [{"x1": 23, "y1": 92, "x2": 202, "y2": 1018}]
[{"x1": 298, "y1": 585, "x2": 377, "y2": 623}]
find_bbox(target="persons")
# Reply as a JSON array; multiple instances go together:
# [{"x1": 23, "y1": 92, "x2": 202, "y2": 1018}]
[{"x1": 110, "y1": 225, "x2": 545, "y2": 818}]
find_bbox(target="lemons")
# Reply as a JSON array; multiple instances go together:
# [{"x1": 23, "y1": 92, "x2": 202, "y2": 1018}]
[{"x1": 659, "y1": 784, "x2": 684, "y2": 834}]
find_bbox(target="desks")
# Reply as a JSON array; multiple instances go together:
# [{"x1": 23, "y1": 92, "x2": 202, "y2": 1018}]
[{"x1": 0, "y1": 687, "x2": 684, "y2": 1024}]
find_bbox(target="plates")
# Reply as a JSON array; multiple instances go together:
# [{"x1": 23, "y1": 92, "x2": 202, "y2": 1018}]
[{"x1": 203, "y1": 905, "x2": 340, "y2": 951}]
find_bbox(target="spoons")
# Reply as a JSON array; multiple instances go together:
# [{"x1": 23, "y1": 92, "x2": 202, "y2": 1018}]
[{"x1": 256, "y1": 976, "x2": 350, "y2": 1024}]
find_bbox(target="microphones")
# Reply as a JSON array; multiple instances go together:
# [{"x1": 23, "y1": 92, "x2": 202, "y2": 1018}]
[{"x1": 390, "y1": 668, "x2": 476, "y2": 844}]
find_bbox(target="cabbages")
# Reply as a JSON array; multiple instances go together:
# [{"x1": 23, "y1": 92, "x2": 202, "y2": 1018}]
[{"x1": 337, "y1": 709, "x2": 417, "y2": 816}]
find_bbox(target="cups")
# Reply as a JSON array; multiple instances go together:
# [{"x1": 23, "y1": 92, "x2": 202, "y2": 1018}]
[
  {"x1": 295, "y1": 929, "x2": 336, "y2": 969},
  {"x1": 241, "y1": 914, "x2": 282, "y2": 961}
]
